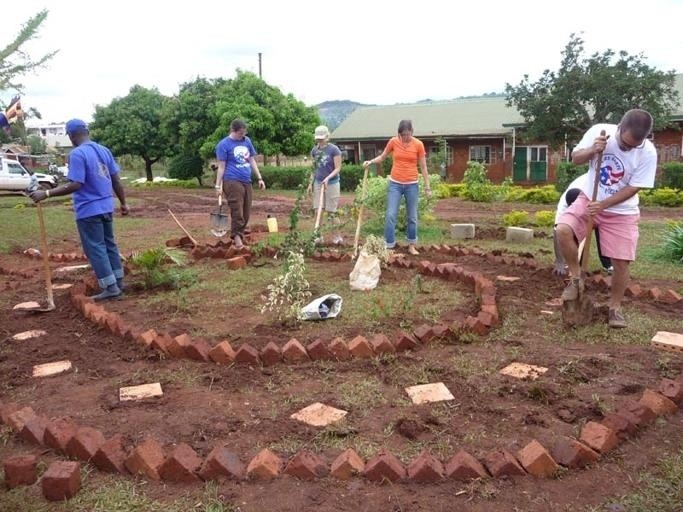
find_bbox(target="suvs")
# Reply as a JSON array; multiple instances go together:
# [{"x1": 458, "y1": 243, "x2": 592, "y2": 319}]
[{"x1": 0, "y1": 156, "x2": 58, "y2": 196}]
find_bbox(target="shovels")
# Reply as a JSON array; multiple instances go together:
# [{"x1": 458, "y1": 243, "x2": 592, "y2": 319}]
[
  {"x1": 561, "y1": 130, "x2": 605, "y2": 328},
  {"x1": 209, "y1": 178, "x2": 228, "y2": 237}
]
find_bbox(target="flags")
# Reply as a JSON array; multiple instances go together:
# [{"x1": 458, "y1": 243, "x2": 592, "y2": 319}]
[{"x1": 6, "y1": 97, "x2": 23, "y2": 124}]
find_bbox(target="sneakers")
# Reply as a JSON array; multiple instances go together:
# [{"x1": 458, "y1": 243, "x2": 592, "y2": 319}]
[
  {"x1": 608, "y1": 307, "x2": 626, "y2": 328},
  {"x1": 235, "y1": 235, "x2": 243, "y2": 248},
  {"x1": 408, "y1": 248, "x2": 419, "y2": 255},
  {"x1": 333, "y1": 236, "x2": 342, "y2": 244},
  {"x1": 563, "y1": 277, "x2": 579, "y2": 301},
  {"x1": 89, "y1": 285, "x2": 120, "y2": 299}
]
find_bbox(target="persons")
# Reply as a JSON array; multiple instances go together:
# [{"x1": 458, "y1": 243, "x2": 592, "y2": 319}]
[
  {"x1": 363, "y1": 120, "x2": 431, "y2": 255},
  {"x1": 53, "y1": 162, "x2": 58, "y2": 176},
  {"x1": 215, "y1": 119, "x2": 265, "y2": 248},
  {"x1": 554, "y1": 109, "x2": 658, "y2": 328},
  {"x1": 0, "y1": 113, "x2": 10, "y2": 136},
  {"x1": 29, "y1": 119, "x2": 129, "y2": 300},
  {"x1": 47, "y1": 162, "x2": 53, "y2": 175},
  {"x1": 307, "y1": 126, "x2": 343, "y2": 245},
  {"x1": 553, "y1": 173, "x2": 615, "y2": 275}
]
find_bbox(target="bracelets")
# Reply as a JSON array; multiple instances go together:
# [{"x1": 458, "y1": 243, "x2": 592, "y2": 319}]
[
  {"x1": 46, "y1": 190, "x2": 49, "y2": 197},
  {"x1": 215, "y1": 186, "x2": 220, "y2": 189},
  {"x1": 257, "y1": 178, "x2": 262, "y2": 180}
]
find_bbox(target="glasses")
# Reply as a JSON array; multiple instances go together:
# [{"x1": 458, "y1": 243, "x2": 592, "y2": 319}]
[{"x1": 619, "y1": 126, "x2": 646, "y2": 150}]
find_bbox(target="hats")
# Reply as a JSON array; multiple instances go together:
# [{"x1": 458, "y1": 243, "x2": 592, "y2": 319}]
[
  {"x1": 315, "y1": 126, "x2": 329, "y2": 139},
  {"x1": 65, "y1": 119, "x2": 86, "y2": 135}
]
